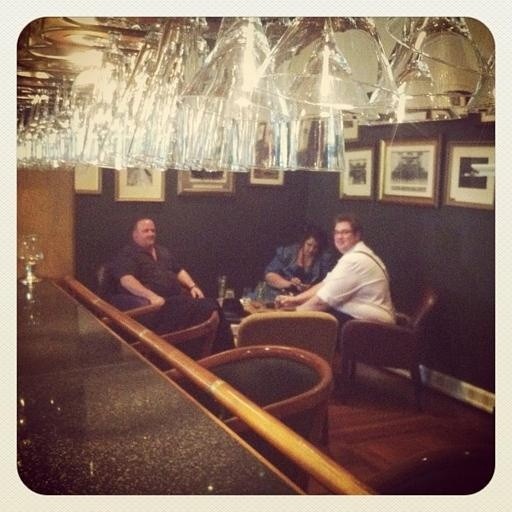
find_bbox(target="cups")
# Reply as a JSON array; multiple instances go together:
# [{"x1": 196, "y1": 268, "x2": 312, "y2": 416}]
[
  {"x1": 254, "y1": 280, "x2": 267, "y2": 301},
  {"x1": 218, "y1": 275, "x2": 229, "y2": 298}
]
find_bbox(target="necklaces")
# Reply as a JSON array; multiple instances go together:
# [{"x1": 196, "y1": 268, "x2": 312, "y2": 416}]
[{"x1": 301, "y1": 248, "x2": 306, "y2": 275}]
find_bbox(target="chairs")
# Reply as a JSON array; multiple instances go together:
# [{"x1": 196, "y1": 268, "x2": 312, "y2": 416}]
[
  {"x1": 161, "y1": 344, "x2": 334, "y2": 491},
  {"x1": 339, "y1": 284, "x2": 449, "y2": 412},
  {"x1": 233, "y1": 311, "x2": 339, "y2": 369},
  {"x1": 99, "y1": 260, "x2": 207, "y2": 306},
  {"x1": 101, "y1": 295, "x2": 232, "y2": 371}
]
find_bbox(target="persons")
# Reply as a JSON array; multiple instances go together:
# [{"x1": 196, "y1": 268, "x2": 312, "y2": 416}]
[
  {"x1": 110, "y1": 213, "x2": 206, "y2": 311},
  {"x1": 272, "y1": 210, "x2": 396, "y2": 350},
  {"x1": 253, "y1": 228, "x2": 336, "y2": 304}
]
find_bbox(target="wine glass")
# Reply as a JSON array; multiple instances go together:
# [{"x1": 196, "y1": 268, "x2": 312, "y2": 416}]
[
  {"x1": 14, "y1": 14, "x2": 496, "y2": 175},
  {"x1": 17, "y1": 231, "x2": 45, "y2": 286},
  {"x1": 20, "y1": 283, "x2": 44, "y2": 326}
]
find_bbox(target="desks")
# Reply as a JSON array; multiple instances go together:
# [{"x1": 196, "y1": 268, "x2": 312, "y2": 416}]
[{"x1": 216, "y1": 296, "x2": 297, "y2": 348}]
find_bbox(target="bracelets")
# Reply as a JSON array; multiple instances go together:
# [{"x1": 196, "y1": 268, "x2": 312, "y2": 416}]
[{"x1": 188, "y1": 281, "x2": 199, "y2": 291}]
